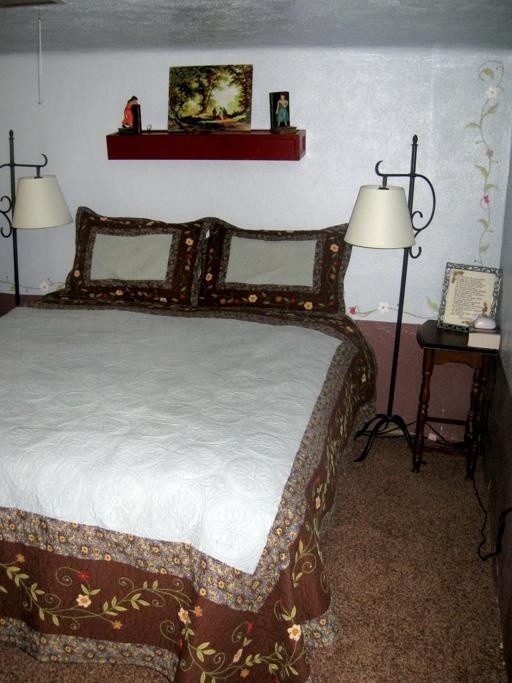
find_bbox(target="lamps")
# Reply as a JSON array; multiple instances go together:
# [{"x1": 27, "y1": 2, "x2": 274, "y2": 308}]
[
  {"x1": 0, "y1": 129, "x2": 73, "y2": 305},
  {"x1": 343, "y1": 135, "x2": 436, "y2": 462}
]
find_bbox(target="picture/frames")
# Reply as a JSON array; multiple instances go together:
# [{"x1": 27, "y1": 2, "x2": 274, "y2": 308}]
[{"x1": 436, "y1": 263, "x2": 503, "y2": 333}]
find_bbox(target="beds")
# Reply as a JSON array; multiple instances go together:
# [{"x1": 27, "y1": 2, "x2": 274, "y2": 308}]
[{"x1": 0, "y1": 289, "x2": 376, "y2": 683}]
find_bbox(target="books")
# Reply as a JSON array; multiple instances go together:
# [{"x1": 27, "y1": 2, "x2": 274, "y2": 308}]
[{"x1": 467, "y1": 324, "x2": 500, "y2": 349}]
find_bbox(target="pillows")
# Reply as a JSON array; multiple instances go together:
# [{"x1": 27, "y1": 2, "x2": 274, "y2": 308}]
[
  {"x1": 195, "y1": 218, "x2": 352, "y2": 320},
  {"x1": 62, "y1": 207, "x2": 211, "y2": 309}
]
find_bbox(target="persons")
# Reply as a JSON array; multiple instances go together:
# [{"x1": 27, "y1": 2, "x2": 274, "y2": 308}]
[
  {"x1": 275, "y1": 93, "x2": 289, "y2": 126},
  {"x1": 130, "y1": 95, "x2": 141, "y2": 128},
  {"x1": 122, "y1": 98, "x2": 140, "y2": 127}
]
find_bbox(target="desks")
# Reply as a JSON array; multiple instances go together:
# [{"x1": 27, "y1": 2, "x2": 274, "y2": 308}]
[{"x1": 411, "y1": 320, "x2": 500, "y2": 481}]
